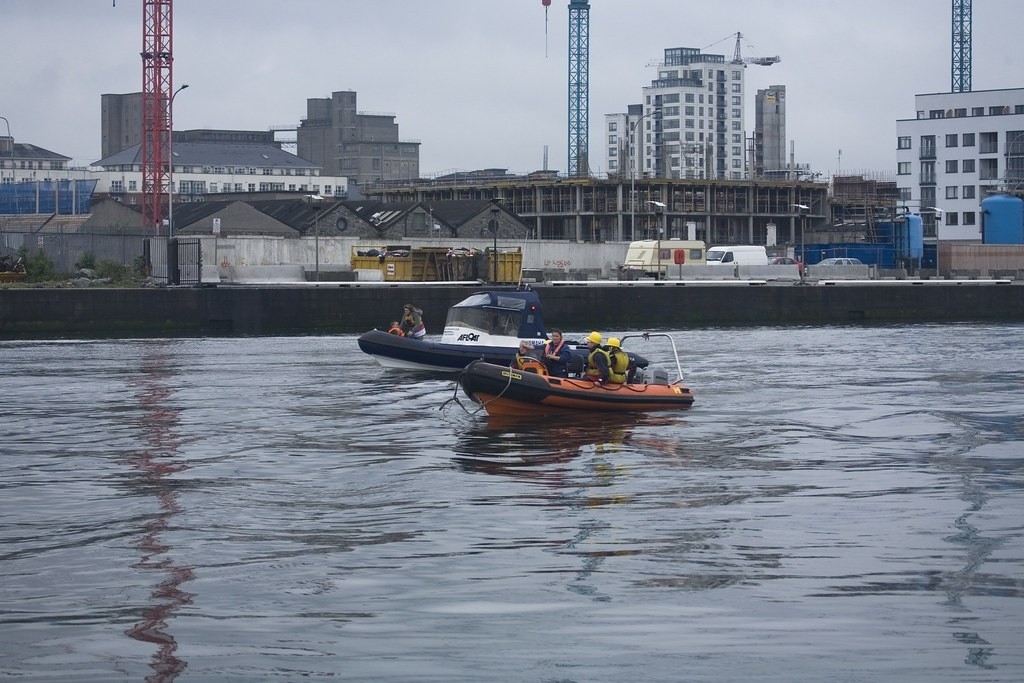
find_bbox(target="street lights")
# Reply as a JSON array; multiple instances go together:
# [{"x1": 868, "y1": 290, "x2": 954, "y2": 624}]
[
  {"x1": 647, "y1": 200, "x2": 666, "y2": 281},
  {"x1": 490, "y1": 197, "x2": 502, "y2": 284},
  {"x1": 429, "y1": 208, "x2": 434, "y2": 238},
  {"x1": 167, "y1": 84, "x2": 190, "y2": 238},
  {"x1": 303, "y1": 193, "x2": 325, "y2": 281},
  {"x1": 789, "y1": 203, "x2": 810, "y2": 281},
  {"x1": 926, "y1": 206, "x2": 944, "y2": 280}
]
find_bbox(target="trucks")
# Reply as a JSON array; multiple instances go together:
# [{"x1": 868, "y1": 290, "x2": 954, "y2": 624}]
[{"x1": 622, "y1": 237, "x2": 707, "y2": 280}]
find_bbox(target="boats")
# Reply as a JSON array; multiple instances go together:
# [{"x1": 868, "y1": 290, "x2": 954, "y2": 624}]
[
  {"x1": 456, "y1": 332, "x2": 696, "y2": 418},
  {"x1": 356, "y1": 280, "x2": 650, "y2": 380},
  {"x1": 0, "y1": 254, "x2": 27, "y2": 283}
]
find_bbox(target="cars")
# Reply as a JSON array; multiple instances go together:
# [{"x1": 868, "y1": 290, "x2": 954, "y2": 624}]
[
  {"x1": 816, "y1": 257, "x2": 864, "y2": 265},
  {"x1": 769, "y1": 257, "x2": 805, "y2": 278}
]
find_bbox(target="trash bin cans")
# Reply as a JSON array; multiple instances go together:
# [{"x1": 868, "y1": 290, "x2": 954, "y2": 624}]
[
  {"x1": 484, "y1": 245, "x2": 522, "y2": 285},
  {"x1": 348, "y1": 242, "x2": 450, "y2": 281},
  {"x1": 449, "y1": 252, "x2": 477, "y2": 281}
]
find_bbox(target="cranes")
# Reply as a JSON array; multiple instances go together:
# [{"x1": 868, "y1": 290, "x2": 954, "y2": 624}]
[{"x1": 645, "y1": 30, "x2": 782, "y2": 69}]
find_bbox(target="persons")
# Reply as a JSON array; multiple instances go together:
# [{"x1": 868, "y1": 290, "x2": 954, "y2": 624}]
[
  {"x1": 510, "y1": 341, "x2": 535, "y2": 370},
  {"x1": 522, "y1": 329, "x2": 571, "y2": 377},
  {"x1": 604, "y1": 338, "x2": 629, "y2": 383},
  {"x1": 583, "y1": 332, "x2": 614, "y2": 386},
  {"x1": 389, "y1": 321, "x2": 404, "y2": 337},
  {"x1": 398, "y1": 304, "x2": 426, "y2": 340}
]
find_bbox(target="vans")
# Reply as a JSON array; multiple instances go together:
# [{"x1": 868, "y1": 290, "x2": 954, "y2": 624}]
[{"x1": 706, "y1": 245, "x2": 769, "y2": 266}]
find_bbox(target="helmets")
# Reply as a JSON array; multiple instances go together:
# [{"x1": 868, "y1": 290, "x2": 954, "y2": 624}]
[
  {"x1": 604, "y1": 338, "x2": 620, "y2": 348},
  {"x1": 586, "y1": 332, "x2": 602, "y2": 344}
]
